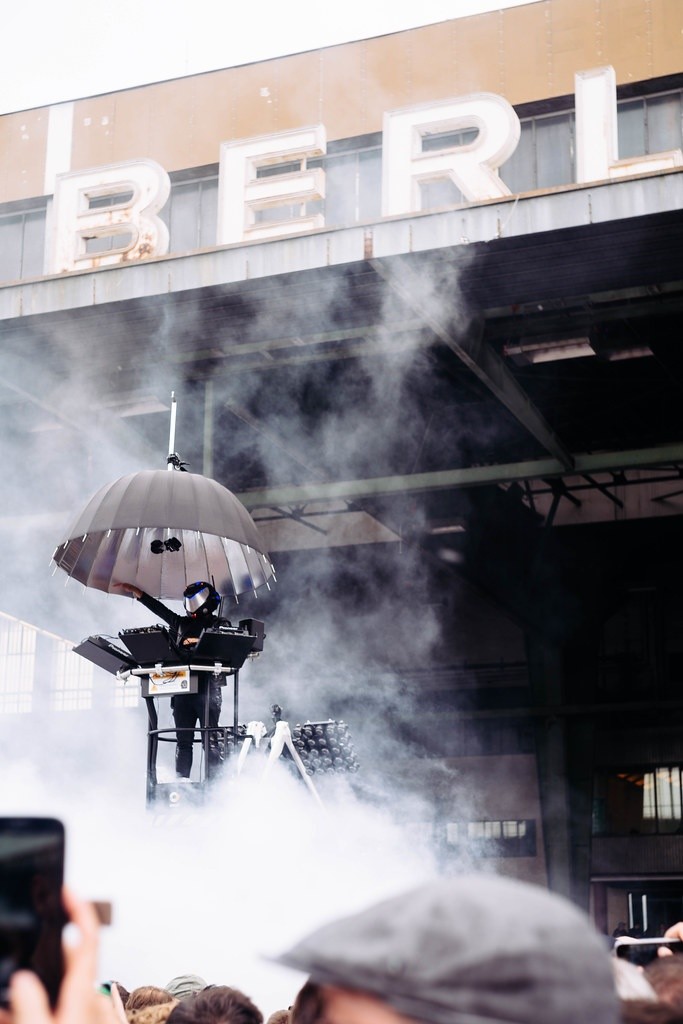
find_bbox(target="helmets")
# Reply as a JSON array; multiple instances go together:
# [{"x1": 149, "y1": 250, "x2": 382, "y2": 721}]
[{"x1": 183, "y1": 581, "x2": 221, "y2": 619}]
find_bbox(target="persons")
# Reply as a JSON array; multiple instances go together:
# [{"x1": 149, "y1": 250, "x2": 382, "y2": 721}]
[
  {"x1": 0, "y1": 881, "x2": 683, "y2": 1024},
  {"x1": 114, "y1": 581, "x2": 233, "y2": 781}
]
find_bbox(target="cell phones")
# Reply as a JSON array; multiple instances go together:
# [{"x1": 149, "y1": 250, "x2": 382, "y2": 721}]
[
  {"x1": 0, "y1": 815, "x2": 66, "y2": 1015},
  {"x1": 613, "y1": 938, "x2": 683, "y2": 967}
]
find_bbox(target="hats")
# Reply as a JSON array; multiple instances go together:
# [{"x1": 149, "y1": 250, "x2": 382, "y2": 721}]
[
  {"x1": 266, "y1": 872, "x2": 620, "y2": 1024},
  {"x1": 164, "y1": 974, "x2": 215, "y2": 1002}
]
